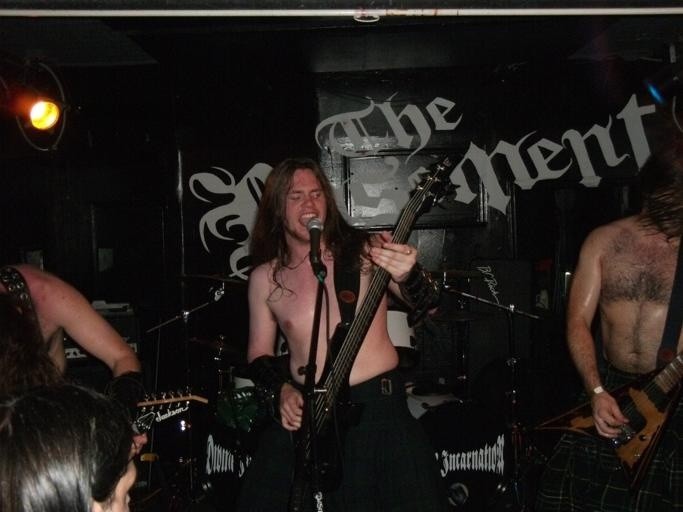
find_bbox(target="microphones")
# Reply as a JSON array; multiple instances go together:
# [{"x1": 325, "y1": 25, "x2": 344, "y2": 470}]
[{"x1": 307, "y1": 218, "x2": 324, "y2": 275}]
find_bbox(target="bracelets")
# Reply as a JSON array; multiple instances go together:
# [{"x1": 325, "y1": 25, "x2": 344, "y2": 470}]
[{"x1": 588, "y1": 385, "x2": 605, "y2": 397}]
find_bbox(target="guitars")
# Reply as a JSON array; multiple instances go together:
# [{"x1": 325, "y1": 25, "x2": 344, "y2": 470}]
[{"x1": 532, "y1": 349, "x2": 683, "y2": 500}]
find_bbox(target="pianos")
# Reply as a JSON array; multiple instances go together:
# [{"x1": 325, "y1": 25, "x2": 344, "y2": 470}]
[{"x1": 63, "y1": 307, "x2": 152, "y2": 370}]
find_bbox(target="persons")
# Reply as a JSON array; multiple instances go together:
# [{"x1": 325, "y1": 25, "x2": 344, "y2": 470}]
[
  {"x1": 0, "y1": 384, "x2": 137, "y2": 512},
  {"x1": 534, "y1": 139, "x2": 682, "y2": 512},
  {"x1": 0, "y1": 259, "x2": 149, "y2": 454}
]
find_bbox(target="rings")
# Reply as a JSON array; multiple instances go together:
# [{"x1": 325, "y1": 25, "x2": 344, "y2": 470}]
[{"x1": 407, "y1": 249, "x2": 412, "y2": 255}]
